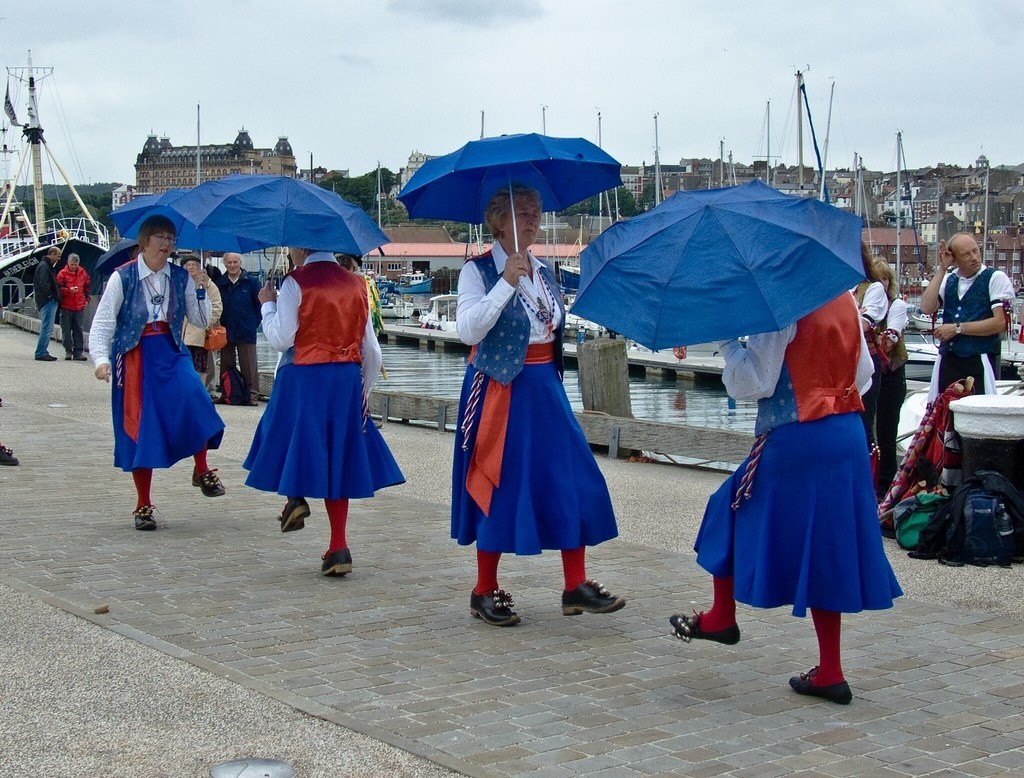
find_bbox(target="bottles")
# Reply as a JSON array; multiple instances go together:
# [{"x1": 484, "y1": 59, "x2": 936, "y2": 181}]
[
  {"x1": 997, "y1": 503, "x2": 1014, "y2": 547},
  {"x1": 577, "y1": 325, "x2": 585, "y2": 346}
]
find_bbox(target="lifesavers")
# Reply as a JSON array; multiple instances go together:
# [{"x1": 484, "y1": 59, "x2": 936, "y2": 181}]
[
  {"x1": 404, "y1": 294, "x2": 411, "y2": 301},
  {"x1": 673, "y1": 346, "x2": 686, "y2": 359}
]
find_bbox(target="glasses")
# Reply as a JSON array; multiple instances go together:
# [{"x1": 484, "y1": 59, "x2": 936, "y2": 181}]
[{"x1": 153, "y1": 234, "x2": 176, "y2": 243}]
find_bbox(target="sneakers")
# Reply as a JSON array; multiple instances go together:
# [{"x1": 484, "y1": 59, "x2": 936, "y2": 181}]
[
  {"x1": 193, "y1": 466, "x2": 225, "y2": 497},
  {"x1": 133, "y1": 504, "x2": 156, "y2": 530}
]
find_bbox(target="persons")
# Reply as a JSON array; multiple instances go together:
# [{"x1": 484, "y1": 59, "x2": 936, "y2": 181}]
[
  {"x1": 850, "y1": 241, "x2": 910, "y2": 499},
  {"x1": 89, "y1": 215, "x2": 226, "y2": 529},
  {"x1": 669, "y1": 293, "x2": 904, "y2": 705},
  {"x1": 451, "y1": 180, "x2": 626, "y2": 626},
  {"x1": 242, "y1": 247, "x2": 406, "y2": 573},
  {"x1": 919, "y1": 232, "x2": 1017, "y2": 409},
  {"x1": 334, "y1": 252, "x2": 384, "y2": 336},
  {"x1": 180, "y1": 249, "x2": 262, "y2": 406},
  {"x1": 58, "y1": 253, "x2": 91, "y2": 361},
  {"x1": 33, "y1": 246, "x2": 62, "y2": 360}
]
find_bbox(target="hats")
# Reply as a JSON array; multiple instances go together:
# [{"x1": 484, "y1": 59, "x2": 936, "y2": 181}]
[{"x1": 180, "y1": 251, "x2": 200, "y2": 268}]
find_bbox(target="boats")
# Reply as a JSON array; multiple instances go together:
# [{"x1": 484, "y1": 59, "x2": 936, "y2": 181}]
[{"x1": 0, "y1": 48, "x2": 113, "y2": 315}]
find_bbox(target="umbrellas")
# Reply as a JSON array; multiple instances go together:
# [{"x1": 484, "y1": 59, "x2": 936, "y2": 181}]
[
  {"x1": 569, "y1": 179, "x2": 868, "y2": 352},
  {"x1": 396, "y1": 132, "x2": 624, "y2": 252},
  {"x1": 107, "y1": 188, "x2": 276, "y2": 299},
  {"x1": 94, "y1": 239, "x2": 142, "y2": 276},
  {"x1": 163, "y1": 172, "x2": 392, "y2": 283}
]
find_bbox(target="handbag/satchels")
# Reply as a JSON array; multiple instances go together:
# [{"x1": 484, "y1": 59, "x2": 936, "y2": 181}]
[
  {"x1": 893, "y1": 491, "x2": 949, "y2": 550},
  {"x1": 204, "y1": 326, "x2": 228, "y2": 351}
]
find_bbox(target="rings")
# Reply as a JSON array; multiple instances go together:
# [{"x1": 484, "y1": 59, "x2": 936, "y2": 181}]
[{"x1": 96, "y1": 375, "x2": 101, "y2": 378}]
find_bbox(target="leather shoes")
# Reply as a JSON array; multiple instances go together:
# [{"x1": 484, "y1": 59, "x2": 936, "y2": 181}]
[
  {"x1": 470, "y1": 587, "x2": 521, "y2": 626},
  {"x1": 562, "y1": 579, "x2": 625, "y2": 615}
]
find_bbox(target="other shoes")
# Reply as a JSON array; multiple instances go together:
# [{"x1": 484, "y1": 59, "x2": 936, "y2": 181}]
[
  {"x1": 250, "y1": 400, "x2": 259, "y2": 406},
  {"x1": 789, "y1": 666, "x2": 853, "y2": 704},
  {"x1": 320, "y1": 549, "x2": 353, "y2": 576},
  {"x1": 65, "y1": 353, "x2": 71, "y2": 359},
  {"x1": 35, "y1": 355, "x2": 57, "y2": 361},
  {"x1": 670, "y1": 609, "x2": 740, "y2": 646},
  {"x1": 73, "y1": 355, "x2": 87, "y2": 360},
  {"x1": 277, "y1": 497, "x2": 311, "y2": 533},
  {"x1": 0, "y1": 446, "x2": 18, "y2": 465}
]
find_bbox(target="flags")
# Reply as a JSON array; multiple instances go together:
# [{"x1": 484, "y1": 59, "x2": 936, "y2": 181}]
[{"x1": 4, "y1": 80, "x2": 23, "y2": 126}]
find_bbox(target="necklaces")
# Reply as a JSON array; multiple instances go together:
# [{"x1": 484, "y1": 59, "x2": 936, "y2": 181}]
[
  {"x1": 517, "y1": 271, "x2": 554, "y2": 338},
  {"x1": 144, "y1": 276, "x2": 168, "y2": 331}
]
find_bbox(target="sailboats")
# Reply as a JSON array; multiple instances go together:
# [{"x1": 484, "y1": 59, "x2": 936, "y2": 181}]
[
  {"x1": 556, "y1": 104, "x2": 668, "y2": 340},
  {"x1": 718, "y1": 62, "x2": 1024, "y2": 383},
  {"x1": 248, "y1": 151, "x2": 485, "y2": 335}
]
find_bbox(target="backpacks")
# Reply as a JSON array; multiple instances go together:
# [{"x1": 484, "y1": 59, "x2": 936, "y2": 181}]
[
  {"x1": 222, "y1": 365, "x2": 250, "y2": 405},
  {"x1": 939, "y1": 469, "x2": 1024, "y2": 566}
]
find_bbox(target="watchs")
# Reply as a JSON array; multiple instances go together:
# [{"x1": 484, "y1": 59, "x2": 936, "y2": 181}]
[{"x1": 955, "y1": 322, "x2": 961, "y2": 334}]
